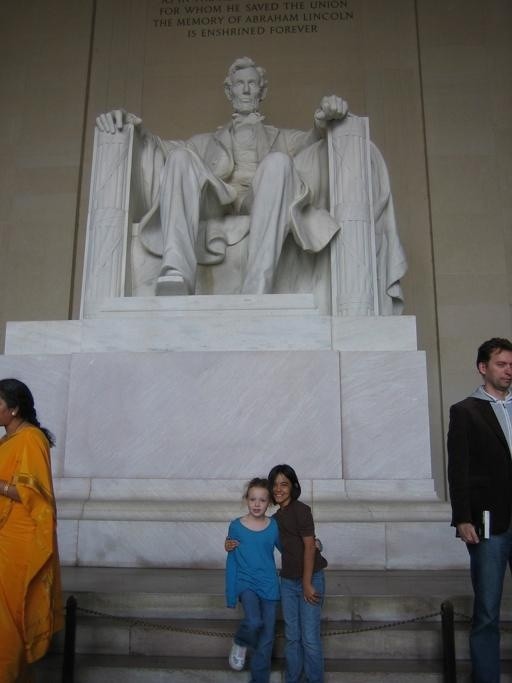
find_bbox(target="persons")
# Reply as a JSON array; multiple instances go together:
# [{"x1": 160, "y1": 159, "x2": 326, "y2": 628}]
[
  {"x1": 448, "y1": 338, "x2": 512, "y2": 683},
  {"x1": 95, "y1": 59, "x2": 350, "y2": 300},
  {"x1": 0, "y1": 378, "x2": 63, "y2": 683}
]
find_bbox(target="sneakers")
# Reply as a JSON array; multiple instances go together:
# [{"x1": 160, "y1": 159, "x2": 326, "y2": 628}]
[{"x1": 226, "y1": 641, "x2": 249, "y2": 671}]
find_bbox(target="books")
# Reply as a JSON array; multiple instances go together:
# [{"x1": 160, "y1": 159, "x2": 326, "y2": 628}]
[{"x1": 456, "y1": 510, "x2": 492, "y2": 538}]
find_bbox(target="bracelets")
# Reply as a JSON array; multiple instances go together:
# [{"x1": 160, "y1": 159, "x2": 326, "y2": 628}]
[{"x1": 3, "y1": 479, "x2": 9, "y2": 497}]
